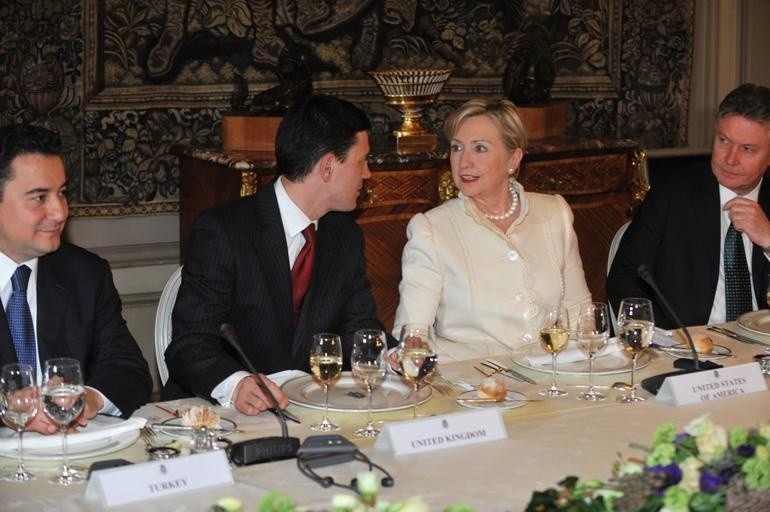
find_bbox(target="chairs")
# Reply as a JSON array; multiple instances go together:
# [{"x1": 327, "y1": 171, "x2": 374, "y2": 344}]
[{"x1": 151, "y1": 267, "x2": 184, "y2": 396}]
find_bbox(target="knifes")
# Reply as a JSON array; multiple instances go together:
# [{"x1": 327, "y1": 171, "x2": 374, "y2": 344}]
[
  {"x1": 659, "y1": 350, "x2": 732, "y2": 357},
  {"x1": 453, "y1": 399, "x2": 544, "y2": 404},
  {"x1": 706, "y1": 326, "x2": 770, "y2": 348},
  {"x1": 149, "y1": 423, "x2": 246, "y2": 433},
  {"x1": 486, "y1": 360, "x2": 538, "y2": 385},
  {"x1": 273, "y1": 404, "x2": 303, "y2": 426}
]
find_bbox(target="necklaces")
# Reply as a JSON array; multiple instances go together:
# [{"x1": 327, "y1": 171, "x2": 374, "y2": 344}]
[{"x1": 484, "y1": 182, "x2": 520, "y2": 220}]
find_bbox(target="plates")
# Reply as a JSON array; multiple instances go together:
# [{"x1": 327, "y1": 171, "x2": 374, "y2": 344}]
[
  {"x1": 159, "y1": 416, "x2": 237, "y2": 440},
  {"x1": 509, "y1": 336, "x2": 652, "y2": 377},
  {"x1": 665, "y1": 341, "x2": 732, "y2": 361},
  {"x1": 279, "y1": 371, "x2": 433, "y2": 414},
  {"x1": 736, "y1": 307, "x2": 770, "y2": 339},
  {"x1": 456, "y1": 388, "x2": 530, "y2": 410},
  {"x1": 0, "y1": 416, "x2": 142, "y2": 461}
]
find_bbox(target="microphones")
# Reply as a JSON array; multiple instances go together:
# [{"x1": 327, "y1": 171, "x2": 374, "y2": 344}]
[
  {"x1": 636, "y1": 263, "x2": 718, "y2": 395},
  {"x1": 220, "y1": 322, "x2": 300, "y2": 466}
]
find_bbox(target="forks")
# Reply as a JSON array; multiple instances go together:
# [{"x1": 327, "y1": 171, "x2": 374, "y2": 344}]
[{"x1": 417, "y1": 375, "x2": 454, "y2": 395}]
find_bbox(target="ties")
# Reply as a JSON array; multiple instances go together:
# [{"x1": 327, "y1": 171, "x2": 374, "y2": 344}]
[
  {"x1": 290, "y1": 222, "x2": 318, "y2": 330},
  {"x1": 5, "y1": 264, "x2": 37, "y2": 388},
  {"x1": 723, "y1": 221, "x2": 753, "y2": 323}
]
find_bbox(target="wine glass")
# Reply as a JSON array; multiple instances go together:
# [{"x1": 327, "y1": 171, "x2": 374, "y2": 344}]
[
  {"x1": 308, "y1": 324, "x2": 438, "y2": 440},
  {"x1": 538, "y1": 297, "x2": 656, "y2": 404},
  {"x1": 0, "y1": 358, "x2": 87, "y2": 487}
]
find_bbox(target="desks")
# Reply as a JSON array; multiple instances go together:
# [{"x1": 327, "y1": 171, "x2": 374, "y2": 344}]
[{"x1": 169, "y1": 143, "x2": 638, "y2": 336}]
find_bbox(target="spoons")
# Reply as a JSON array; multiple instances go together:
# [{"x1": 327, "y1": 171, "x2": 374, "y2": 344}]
[
  {"x1": 568, "y1": 381, "x2": 636, "y2": 394},
  {"x1": 139, "y1": 428, "x2": 180, "y2": 459}
]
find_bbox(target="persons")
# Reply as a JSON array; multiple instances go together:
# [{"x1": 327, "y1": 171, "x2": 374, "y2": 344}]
[
  {"x1": 159, "y1": 94, "x2": 430, "y2": 417},
  {"x1": 603, "y1": 83, "x2": 770, "y2": 336},
  {"x1": 0, "y1": 121, "x2": 155, "y2": 436},
  {"x1": 389, "y1": 97, "x2": 596, "y2": 367}
]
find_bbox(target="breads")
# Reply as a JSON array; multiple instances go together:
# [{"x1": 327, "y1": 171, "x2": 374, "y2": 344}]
[
  {"x1": 182, "y1": 404, "x2": 220, "y2": 432},
  {"x1": 476, "y1": 376, "x2": 510, "y2": 403},
  {"x1": 688, "y1": 333, "x2": 713, "y2": 356}
]
